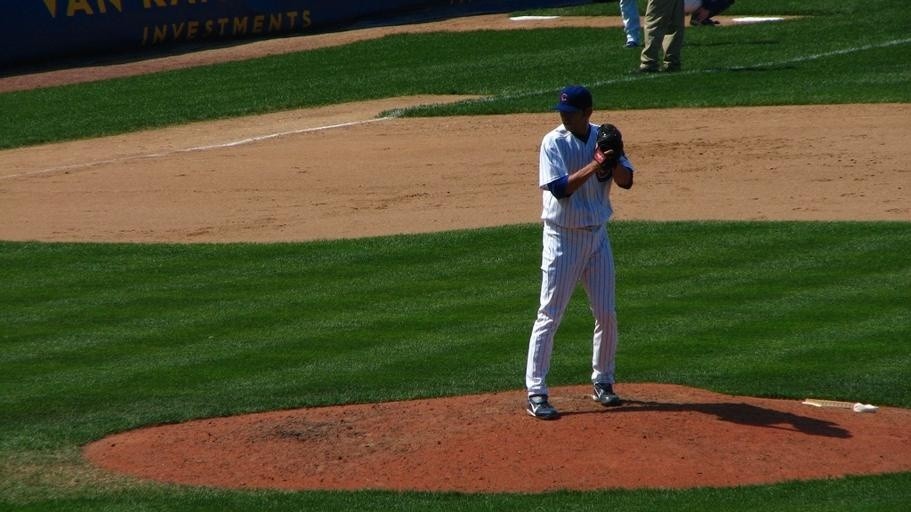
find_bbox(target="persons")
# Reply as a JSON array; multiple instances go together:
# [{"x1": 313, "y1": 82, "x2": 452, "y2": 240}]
[
  {"x1": 683, "y1": 1, "x2": 739, "y2": 28},
  {"x1": 525, "y1": 82, "x2": 636, "y2": 422},
  {"x1": 637, "y1": 0, "x2": 685, "y2": 74},
  {"x1": 620, "y1": 1, "x2": 642, "y2": 48}
]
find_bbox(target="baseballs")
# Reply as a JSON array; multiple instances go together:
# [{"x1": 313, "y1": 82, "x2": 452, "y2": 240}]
[{"x1": 854, "y1": 402, "x2": 865, "y2": 413}]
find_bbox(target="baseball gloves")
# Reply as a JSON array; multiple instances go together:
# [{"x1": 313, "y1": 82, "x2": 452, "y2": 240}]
[{"x1": 595, "y1": 124, "x2": 622, "y2": 168}]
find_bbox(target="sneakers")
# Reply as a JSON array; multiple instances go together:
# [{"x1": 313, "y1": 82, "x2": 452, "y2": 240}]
[
  {"x1": 624, "y1": 41, "x2": 637, "y2": 48},
  {"x1": 526, "y1": 394, "x2": 558, "y2": 420},
  {"x1": 592, "y1": 382, "x2": 619, "y2": 406}
]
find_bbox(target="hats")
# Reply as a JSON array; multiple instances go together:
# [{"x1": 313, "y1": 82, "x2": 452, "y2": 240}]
[{"x1": 556, "y1": 84, "x2": 593, "y2": 112}]
